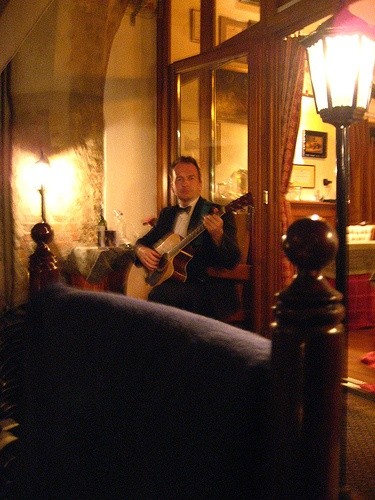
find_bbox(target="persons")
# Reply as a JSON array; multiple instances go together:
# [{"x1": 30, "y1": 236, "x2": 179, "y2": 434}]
[{"x1": 133, "y1": 156, "x2": 242, "y2": 322}]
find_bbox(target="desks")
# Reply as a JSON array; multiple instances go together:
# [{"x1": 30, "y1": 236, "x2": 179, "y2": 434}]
[
  {"x1": 321, "y1": 244, "x2": 375, "y2": 331},
  {"x1": 67, "y1": 246, "x2": 135, "y2": 296}
]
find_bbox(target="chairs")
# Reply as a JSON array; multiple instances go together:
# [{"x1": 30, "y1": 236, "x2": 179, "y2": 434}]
[
  {"x1": 345, "y1": 224, "x2": 375, "y2": 242},
  {"x1": 0, "y1": 216, "x2": 345, "y2": 500}
]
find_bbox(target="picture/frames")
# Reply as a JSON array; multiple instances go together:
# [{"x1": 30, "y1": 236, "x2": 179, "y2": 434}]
[
  {"x1": 301, "y1": 130, "x2": 328, "y2": 158},
  {"x1": 191, "y1": 9, "x2": 200, "y2": 43},
  {"x1": 287, "y1": 164, "x2": 316, "y2": 189},
  {"x1": 181, "y1": 120, "x2": 222, "y2": 166},
  {"x1": 219, "y1": 16, "x2": 249, "y2": 64}
]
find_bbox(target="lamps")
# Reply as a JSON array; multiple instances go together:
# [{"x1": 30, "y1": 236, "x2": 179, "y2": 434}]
[{"x1": 299, "y1": 6, "x2": 375, "y2": 500}]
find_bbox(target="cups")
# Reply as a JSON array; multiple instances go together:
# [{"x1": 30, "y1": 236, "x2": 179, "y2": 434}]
[{"x1": 106, "y1": 231, "x2": 116, "y2": 247}]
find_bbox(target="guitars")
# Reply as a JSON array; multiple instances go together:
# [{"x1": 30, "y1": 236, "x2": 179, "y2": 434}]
[{"x1": 126, "y1": 192, "x2": 256, "y2": 303}]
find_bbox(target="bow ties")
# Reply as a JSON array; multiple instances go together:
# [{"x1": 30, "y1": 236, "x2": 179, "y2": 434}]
[{"x1": 177, "y1": 206, "x2": 192, "y2": 215}]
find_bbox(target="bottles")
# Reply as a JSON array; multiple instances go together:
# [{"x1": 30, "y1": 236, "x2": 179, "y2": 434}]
[{"x1": 97, "y1": 209, "x2": 107, "y2": 247}]
